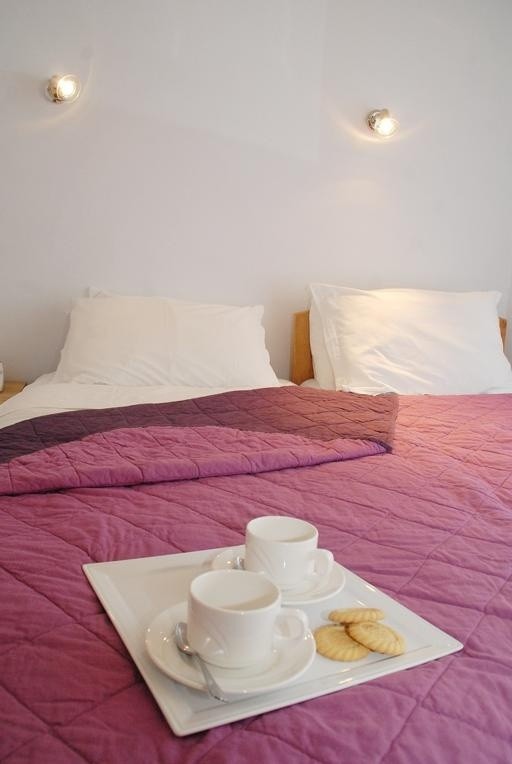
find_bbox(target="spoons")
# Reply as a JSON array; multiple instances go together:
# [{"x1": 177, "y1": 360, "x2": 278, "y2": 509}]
[{"x1": 174, "y1": 620, "x2": 229, "y2": 704}]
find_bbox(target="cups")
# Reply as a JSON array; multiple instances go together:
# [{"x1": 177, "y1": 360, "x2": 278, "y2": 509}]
[
  {"x1": 244, "y1": 515, "x2": 334, "y2": 591},
  {"x1": 81, "y1": 544, "x2": 464, "y2": 739},
  {"x1": 186, "y1": 568, "x2": 310, "y2": 670}
]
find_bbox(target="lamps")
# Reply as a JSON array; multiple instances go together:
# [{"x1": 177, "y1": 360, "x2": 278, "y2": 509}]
[
  {"x1": 45, "y1": 73, "x2": 82, "y2": 104},
  {"x1": 366, "y1": 108, "x2": 400, "y2": 137}
]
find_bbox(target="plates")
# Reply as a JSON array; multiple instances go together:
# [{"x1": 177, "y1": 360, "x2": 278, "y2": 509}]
[
  {"x1": 145, "y1": 599, "x2": 317, "y2": 696},
  {"x1": 213, "y1": 545, "x2": 345, "y2": 607}
]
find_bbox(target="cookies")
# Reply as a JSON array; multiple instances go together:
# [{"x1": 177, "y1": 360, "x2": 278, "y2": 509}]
[
  {"x1": 328, "y1": 607, "x2": 383, "y2": 623},
  {"x1": 313, "y1": 625, "x2": 370, "y2": 662},
  {"x1": 347, "y1": 621, "x2": 405, "y2": 656}
]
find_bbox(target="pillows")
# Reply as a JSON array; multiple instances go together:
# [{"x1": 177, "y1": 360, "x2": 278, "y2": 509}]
[
  {"x1": 309, "y1": 279, "x2": 512, "y2": 396},
  {"x1": 47, "y1": 285, "x2": 282, "y2": 388}
]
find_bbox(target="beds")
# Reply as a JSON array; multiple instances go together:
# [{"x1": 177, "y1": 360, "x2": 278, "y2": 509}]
[{"x1": 0, "y1": 311, "x2": 512, "y2": 764}]
